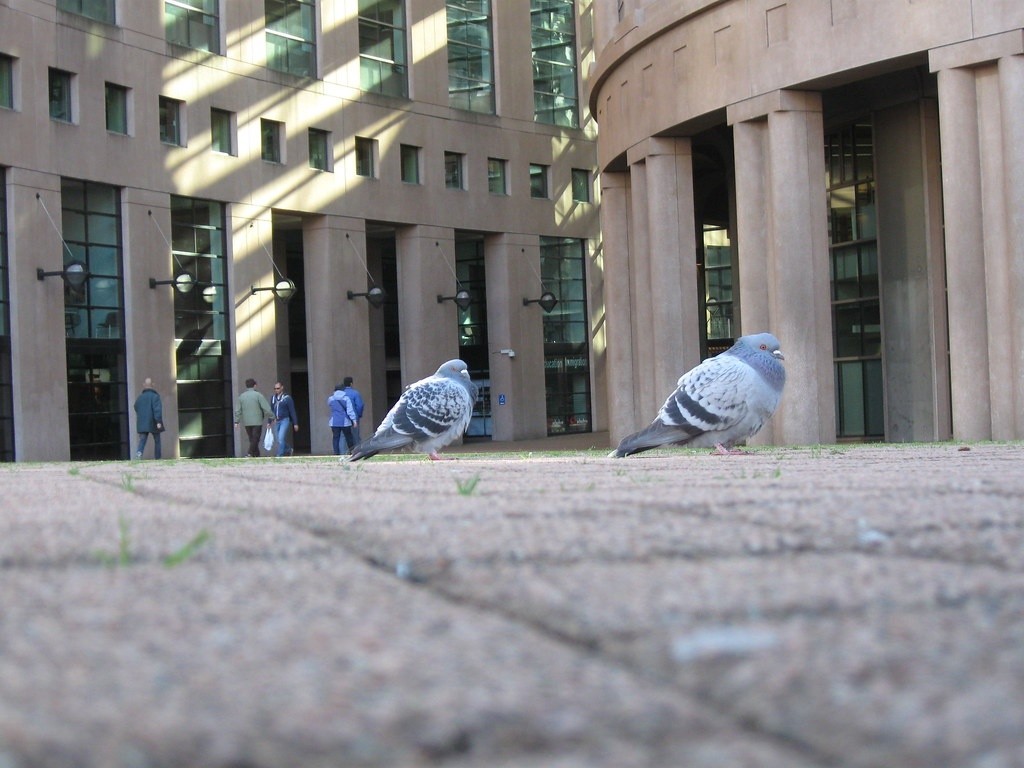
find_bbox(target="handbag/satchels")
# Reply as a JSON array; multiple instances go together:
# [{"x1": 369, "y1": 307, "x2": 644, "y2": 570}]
[{"x1": 264, "y1": 428, "x2": 273, "y2": 450}]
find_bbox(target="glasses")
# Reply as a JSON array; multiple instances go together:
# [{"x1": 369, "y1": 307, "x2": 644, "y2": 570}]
[{"x1": 274, "y1": 387, "x2": 282, "y2": 390}]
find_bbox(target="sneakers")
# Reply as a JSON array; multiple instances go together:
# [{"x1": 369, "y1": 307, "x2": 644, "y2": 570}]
[{"x1": 288, "y1": 448, "x2": 293, "y2": 456}]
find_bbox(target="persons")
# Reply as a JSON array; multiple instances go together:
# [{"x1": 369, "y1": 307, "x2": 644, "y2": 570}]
[
  {"x1": 134, "y1": 378, "x2": 166, "y2": 459},
  {"x1": 326, "y1": 382, "x2": 358, "y2": 456},
  {"x1": 234, "y1": 378, "x2": 276, "y2": 457},
  {"x1": 266, "y1": 381, "x2": 299, "y2": 456},
  {"x1": 338, "y1": 377, "x2": 364, "y2": 455}
]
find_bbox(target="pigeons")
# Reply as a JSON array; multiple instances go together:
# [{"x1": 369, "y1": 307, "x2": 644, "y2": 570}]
[
  {"x1": 343, "y1": 358, "x2": 479, "y2": 462},
  {"x1": 607, "y1": 332, "x2": 786, "y2": 454}
]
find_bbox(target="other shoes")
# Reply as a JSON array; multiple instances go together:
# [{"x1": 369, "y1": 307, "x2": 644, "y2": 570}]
[
  {"x1": 136, "y1": 456, "x2": 142, "y2": 460},
  {"x1": 245, "y1": 453, "x2": 251, "y2": 457}
]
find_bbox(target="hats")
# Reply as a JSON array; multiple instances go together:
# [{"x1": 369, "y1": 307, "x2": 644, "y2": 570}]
[{"x1": 335, "y1": 385, "x2": 344, "y2": 390}]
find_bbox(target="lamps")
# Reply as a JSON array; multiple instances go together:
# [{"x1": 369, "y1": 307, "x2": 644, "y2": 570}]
[
  {"x1": 437, "y1": 290, "x2": 472, "y2": 311},
  {"x1": 150, "y1": 271, "x2": 197, "y2": 293},
  {"x1": 37, "y1": 261, "x2": 91, "y2": 293},
  {"x1": 347, "y1": 285, "x2": 386, "y2": 308},
  {"x1": 252, "y1": 278, "x2": 296, "y2": 300},
  {"x1": 523, "y1": 292, "x2": 558, "y2": 313}
]
